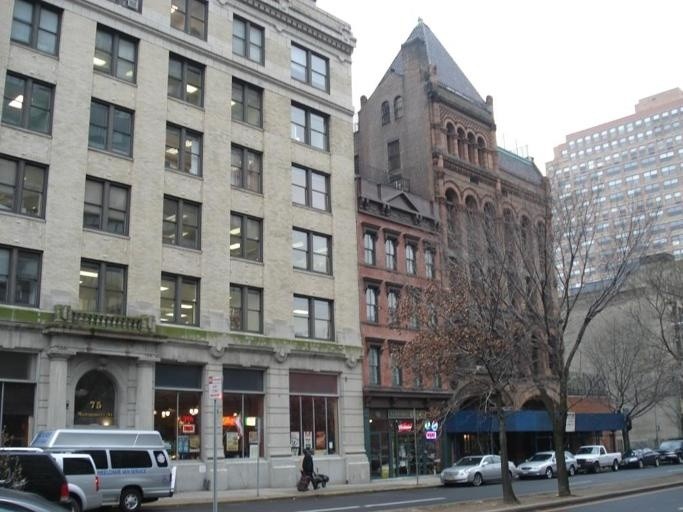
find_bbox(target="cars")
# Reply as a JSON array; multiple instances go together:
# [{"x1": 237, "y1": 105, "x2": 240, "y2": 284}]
[{"x1": 433, "y1": 435, "x2": 682, "y2": 486}]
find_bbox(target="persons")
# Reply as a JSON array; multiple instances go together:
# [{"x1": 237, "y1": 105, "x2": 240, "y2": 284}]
[{"x1": 301, "y1": 448, "x2": 320, "y2": 492}]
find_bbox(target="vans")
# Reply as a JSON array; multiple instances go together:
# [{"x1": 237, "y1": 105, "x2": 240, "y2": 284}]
[{"x1": 0, "y1": 427, "x2": 180, "y2": 510}]
